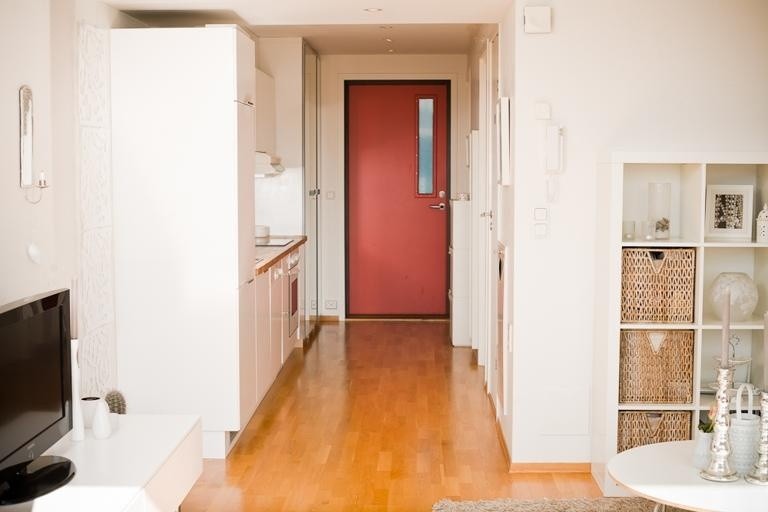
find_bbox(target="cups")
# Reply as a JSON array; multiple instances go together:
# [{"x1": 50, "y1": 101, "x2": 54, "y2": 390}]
[{"x1": 622, "y1": 182, "x2": 671, "y2": 241}]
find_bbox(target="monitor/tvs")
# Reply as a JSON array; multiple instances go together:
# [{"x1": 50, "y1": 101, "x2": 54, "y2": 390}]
[{"x1": 1, "y1": 287, "x2": 75, "y2": 505}]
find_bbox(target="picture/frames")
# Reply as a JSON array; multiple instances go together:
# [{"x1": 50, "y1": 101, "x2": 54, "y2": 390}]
[{"x1": 706, "y1": 184, "x2": 755, "y2": 241}]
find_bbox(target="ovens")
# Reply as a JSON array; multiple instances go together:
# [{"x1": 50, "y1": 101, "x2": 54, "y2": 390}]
[{"x1": 280, "y1": 249, "x2": 298, "y2": 366}]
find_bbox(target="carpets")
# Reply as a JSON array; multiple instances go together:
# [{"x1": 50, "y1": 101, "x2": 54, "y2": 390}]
[{"x1": 434, "y1": 497, "x2": 667, "y2": 512}]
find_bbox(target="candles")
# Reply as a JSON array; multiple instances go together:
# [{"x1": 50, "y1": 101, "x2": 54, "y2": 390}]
[{"x1": 720, "y1": 289, "x2": 731, "y2": 368}]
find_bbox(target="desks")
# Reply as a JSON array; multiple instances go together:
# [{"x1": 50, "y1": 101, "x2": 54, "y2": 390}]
[{"x1": 0, "y1": 397, "x2": 209, "y2": 512}]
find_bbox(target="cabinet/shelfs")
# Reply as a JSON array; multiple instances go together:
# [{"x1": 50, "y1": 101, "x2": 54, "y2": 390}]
[
  {"x1": 258, "y1": 262, "x2": 281, "y2": 404},
  {"x1": 594, "y1": 154, "x2": 768, "y2": 498},
  {"x1": 254, "y1": 38, "x2": 319, "y2": 344},
  {"x1": 109, "y1": 26, "x2": 258, "y2": 458},
  {"x1": 448, "y1": 198, "x2": 469, "y2": 346}
]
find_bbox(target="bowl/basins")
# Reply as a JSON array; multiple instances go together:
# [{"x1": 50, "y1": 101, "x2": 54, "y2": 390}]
[{"x1": 257, "y1": 224, "x2": 270, "y2": 238}]
